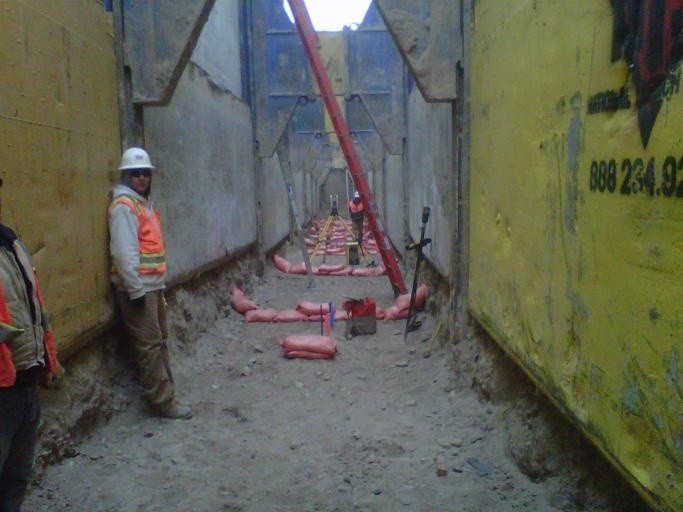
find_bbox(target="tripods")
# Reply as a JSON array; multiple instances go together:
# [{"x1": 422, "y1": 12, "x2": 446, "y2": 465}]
[{"x1": 309, "y1": 209, "x2": 375, "y2": 269}]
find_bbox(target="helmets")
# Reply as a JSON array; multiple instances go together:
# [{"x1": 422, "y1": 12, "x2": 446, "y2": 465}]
[
  {"x1": 354, "y1": 191, "x2": 359, "y2": 197},
  {"x1": 118, "y1": 147, "x2": 155, "y2": 170}
]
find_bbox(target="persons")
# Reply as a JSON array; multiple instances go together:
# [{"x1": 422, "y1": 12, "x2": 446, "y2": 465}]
[
  {"x1": 109, "y1": 148, "x2": 192, "y2": 419},
  {"x1": 0, "y1": 177, "x2": 60, "y2": 512},
  {"x1": 349, "y1": 192, "x2": 364, "y2": 242}
]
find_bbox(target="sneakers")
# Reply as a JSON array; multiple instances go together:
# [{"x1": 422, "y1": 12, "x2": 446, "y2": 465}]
[{"x1": 162, "y1": 399, "x2": 191, "y2": 419}]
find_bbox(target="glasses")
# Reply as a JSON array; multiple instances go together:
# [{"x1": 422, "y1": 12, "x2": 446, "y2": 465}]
[{"x1": 131, "y1": 169, "x2": 150, "y2": 177}]
